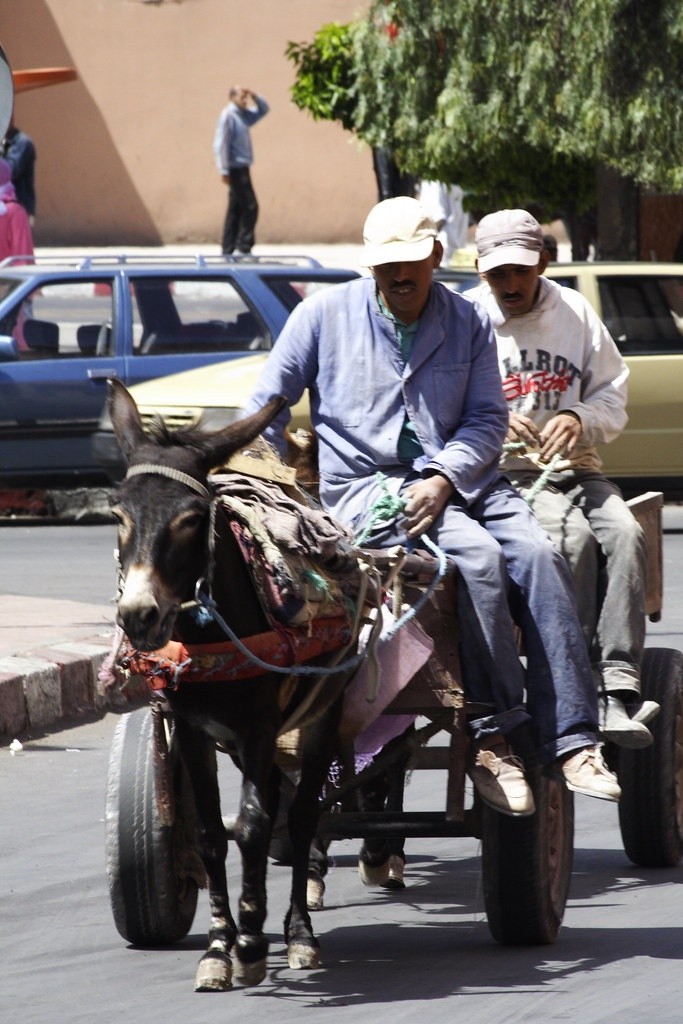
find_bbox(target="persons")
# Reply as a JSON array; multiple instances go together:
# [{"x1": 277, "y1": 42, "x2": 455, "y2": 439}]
[
  {"x1": 5, "y1": 127, "x2": 35, "y2": 256},
  {"x1": 245, "y1": 195, "x2": 623, "y2": 818},
  {"x1": 457, "y1": 206, "x2": 661, "y2": 748},
  {"x1": 0, "y1": 157, "x2": 40, "y2": 352},
  {"x1": 214, "y1": 87, "x2": 269, "y2": 263}
]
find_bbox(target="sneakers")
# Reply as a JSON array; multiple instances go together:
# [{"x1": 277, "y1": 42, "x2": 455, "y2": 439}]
[
  {"x1": 625, "y1": 699, "x2": 661, "y2": 722},
  {"x1": 544, "y1": 741, "x2": 622, "y2": 802},
  {"x1": 308, "y1": 880, "x2": 326, "y2": 910},
  {"x1": 597, "y1": 696, "x2": 652, "y2": 749},
  {"x1": 465, "y1": 734, "x2": 537, "y2": 816}
]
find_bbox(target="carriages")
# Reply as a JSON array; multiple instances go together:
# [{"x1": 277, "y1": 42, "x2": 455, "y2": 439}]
[{"x1": 92, "y1": 373, "x2": 683, "y2": 949}]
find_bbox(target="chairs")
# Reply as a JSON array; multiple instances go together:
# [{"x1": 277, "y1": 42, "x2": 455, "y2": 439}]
[
  {"x1": 17, "y1": 318, "x2": 60, "y2": 359},
  {"x1": 75, "y1": 323, "x2": 110, "y2": 357}
]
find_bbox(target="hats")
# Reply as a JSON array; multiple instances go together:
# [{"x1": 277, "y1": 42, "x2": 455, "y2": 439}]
[
  {"x1": 359, "y1": 196, "x2": 440, "y2": 268},
  {"x1": 474, "y1": 208, "x2": 543, "y2": 274}
]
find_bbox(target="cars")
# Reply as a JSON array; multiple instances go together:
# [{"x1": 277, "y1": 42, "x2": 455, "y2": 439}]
[{"x1": 438, "y1": 262, "x2": 683, "y2": 493}]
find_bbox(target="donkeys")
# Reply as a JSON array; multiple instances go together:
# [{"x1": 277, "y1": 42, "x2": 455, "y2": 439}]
[{"x1": 105, "y1": 374, "x2": 408, "y2": 994}]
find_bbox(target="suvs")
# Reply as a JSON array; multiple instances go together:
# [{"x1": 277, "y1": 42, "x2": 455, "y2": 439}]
[{"x1": 0, "y1": 252, "x2": 364, "y2": 491}]
